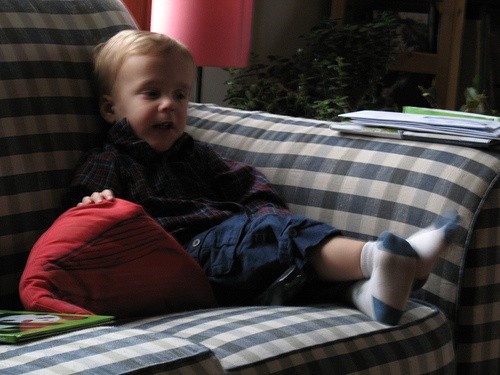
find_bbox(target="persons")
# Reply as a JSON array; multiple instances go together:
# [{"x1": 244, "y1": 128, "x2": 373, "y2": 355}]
[{"x1": 56, "y1": 29, "x2": 463, "y2": 327}]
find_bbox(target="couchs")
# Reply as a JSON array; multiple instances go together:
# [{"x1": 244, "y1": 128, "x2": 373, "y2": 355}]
[{"x1": 0, "y1": 0, "x2": 500, "y2": 375}]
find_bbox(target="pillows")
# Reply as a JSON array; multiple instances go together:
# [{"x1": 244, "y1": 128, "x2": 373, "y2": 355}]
[{"x1": 19, "y1": 196, "x2": 218, "y2": 315}]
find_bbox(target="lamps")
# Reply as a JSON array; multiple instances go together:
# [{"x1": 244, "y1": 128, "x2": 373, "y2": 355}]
[{"x1": 149, "y1": 0, "x2": 253, "y2": 103}]
